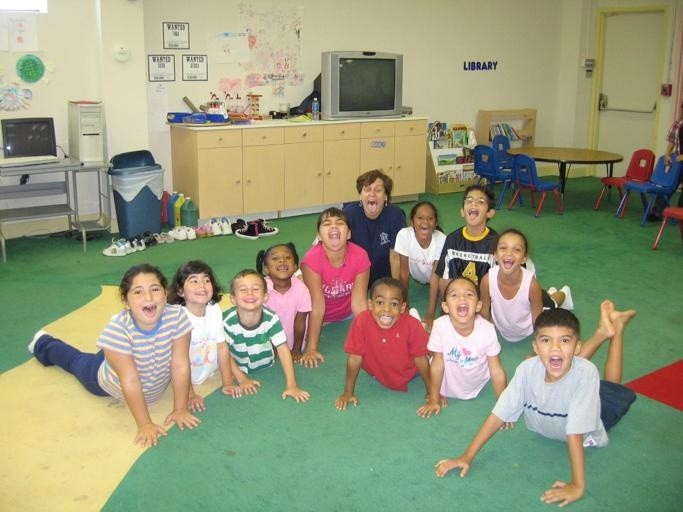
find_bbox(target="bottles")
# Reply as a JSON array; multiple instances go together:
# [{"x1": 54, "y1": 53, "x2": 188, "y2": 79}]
[
  {"x1": 456, "y1": 148, "x2": 474, "y2": 163},
  {"x1": 448, "y1": 128, "x2": 467, "y2": 146},
  {"x1": 208, "y1": 92, "x2": 242, "y2": 113},
  {"x1": 311, "y1": 97, "x2": 319, "y2": 120}
]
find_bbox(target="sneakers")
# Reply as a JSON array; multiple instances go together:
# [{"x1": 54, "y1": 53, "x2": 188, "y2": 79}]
[
  {"x1": 217, "y1": 217, "x2": 232, "y2": 236},
  {"x1": 192, "y1": 226, "x2": 206, "y2": 238},
  {"x1": 167, "y1": 227, "x2": 187, "y2": 240},
  {"x1": 142, "y1": 231, "x2": 156, "y2": 246},
  {"x1": 181, "y1": 226, "x2": 197, "y2": 240},
  {"x1": 202, "y1": 224, "x2": 213, "y2": 238},
  {"x1": 152, "y1": 233, "x2": 164, "y2": 243},
  {"x1": 230, "y1": 219, "x2": 245, "y2": 235},
  {"x1": 141, "y1": 235, "x2": 152, "y2": 248},
  {"x1": 130, "y1": 235, "x2": 145, "y2": 251},
  {"x1": 102, "y1": 243, "x2": 126, "y2": 256},
  {"x1": 116, "y1": 238, "x2": 131, "y2": 254},
  {"x1": 207, "y1": 218, "x2": 222, "y2": 236},
  {"x1": 120, "y1": 237, "x2": 136, "y2": 252},
  {"x1": 159, "y1": 232, "x2": 174, "y2": 243}
]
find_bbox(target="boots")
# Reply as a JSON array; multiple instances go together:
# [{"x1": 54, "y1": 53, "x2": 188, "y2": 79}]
[
  {"x1": 254, "y1": 219, "x2": 279, "y2": 237},
  {"x1": 234, "y1": 222, "x2": 259, "y2": 240}
]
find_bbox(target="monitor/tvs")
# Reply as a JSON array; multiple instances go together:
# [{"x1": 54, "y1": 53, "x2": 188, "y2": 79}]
[
  {"x1": 321, "y1": 51, "x2": 403, "y2": 120},
  {"x1": 0, "y1": 117, "x2": 58, "y2": 167}
]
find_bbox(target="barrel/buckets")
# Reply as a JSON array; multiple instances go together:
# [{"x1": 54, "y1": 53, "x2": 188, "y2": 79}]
[{"x1": 162, "y1": 189, "x2": 197, "y2": 227}]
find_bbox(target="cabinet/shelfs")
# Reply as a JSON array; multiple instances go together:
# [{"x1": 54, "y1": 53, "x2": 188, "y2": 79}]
[
  {"x1": 168, "y1": 126, "x2": 283, "y2": 230},
  {"x1": 425, "y1": 123, "x2": 484, "y2": 195},
  {"x1": 283, "y1": 123, "x2": 361, "y2": 219},
  {"x1": 360, "y1": 118, "x2": 430, "y2": 204},
  {"x1": 476, "y1": 106, "x2": 537, "y2": 150},
  {"x1": 66, "y1": 163, "x2": 112, "y2": 237},
  {"x1": 0, "y1": 155, "x2": 89, "y2": 264}
]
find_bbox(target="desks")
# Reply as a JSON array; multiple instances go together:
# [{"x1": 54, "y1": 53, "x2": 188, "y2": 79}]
[{"x1": 503, "y1": 145, "x2": 625, "y2": 212}]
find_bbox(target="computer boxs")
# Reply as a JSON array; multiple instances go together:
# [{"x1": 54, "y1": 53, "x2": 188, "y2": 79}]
[{"x1": 68, "y1": 100, "x2": 108, "y2": 166}]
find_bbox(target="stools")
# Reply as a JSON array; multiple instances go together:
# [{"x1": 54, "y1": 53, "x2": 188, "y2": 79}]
[{"x1": 649, "y1": 206, "x2": 682, "y2": 249}]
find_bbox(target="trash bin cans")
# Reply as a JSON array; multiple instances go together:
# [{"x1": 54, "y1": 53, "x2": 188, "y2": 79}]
[{"x1": 107, "y1": 150, "x2": 165, "y2": 241}]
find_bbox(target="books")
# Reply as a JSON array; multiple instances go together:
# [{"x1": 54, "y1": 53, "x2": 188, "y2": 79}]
[{"x1": 489, "y1": 123, "x2": 523, "y2": 142}]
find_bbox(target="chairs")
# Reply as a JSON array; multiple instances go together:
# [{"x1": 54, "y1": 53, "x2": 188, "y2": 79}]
[
  {"x1": 507, "y1": 154, "x2": 564, "y2": 218},
  {"x1": 615, "y1": 152, "x2": 683, "y2": 227},
  {"x1": 491, "y1": 134, "x2": 518, "y2": 169},
  {"x1": 593, "y1": 148, "x2": 656, "y2": 219},
  {"x1": 473, "y1": 144, "x2": 524, "y2": 210}
]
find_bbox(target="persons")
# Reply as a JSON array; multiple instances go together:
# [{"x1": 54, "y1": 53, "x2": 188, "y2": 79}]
[
  {"x1": 256, "y1": 241, "x2": 313, "y2": 363},
  {"x1": 28, "y1": 263, "x2": 201, "y2": 447},
  {"x1": 648, "y1": 103, "x2": 683, "y2": 224},
  {"x1": 222, "y1": 268, "x2": 311, "y2": 405},
  {"x1": 341, "y1": 170, "x2": 407, "y2": 282},
  {"x1": 300, "y1": 208, "x2": 371, "y2": 367},
  {"x1": 434, "y1": 300, "x2": 636, "y2": 508},
  {"x1": 479, "y1": 229, "x2": 574, "y2": 361},
  {"x1": 334, "y1": 277, "x2": 448, "y2": 412},
  {"x1": 434, "y1": 183, "x2": 500, "y2": 306},
  {"x1": 168, "y1": 257, "x2": 244, "y2": 412},
  {"x1": 416, "y1": 276, "x2": 517, "y2": 432},
  {"x1": 393, "y1": 201, "x2": 448, "y2": 326}
]
